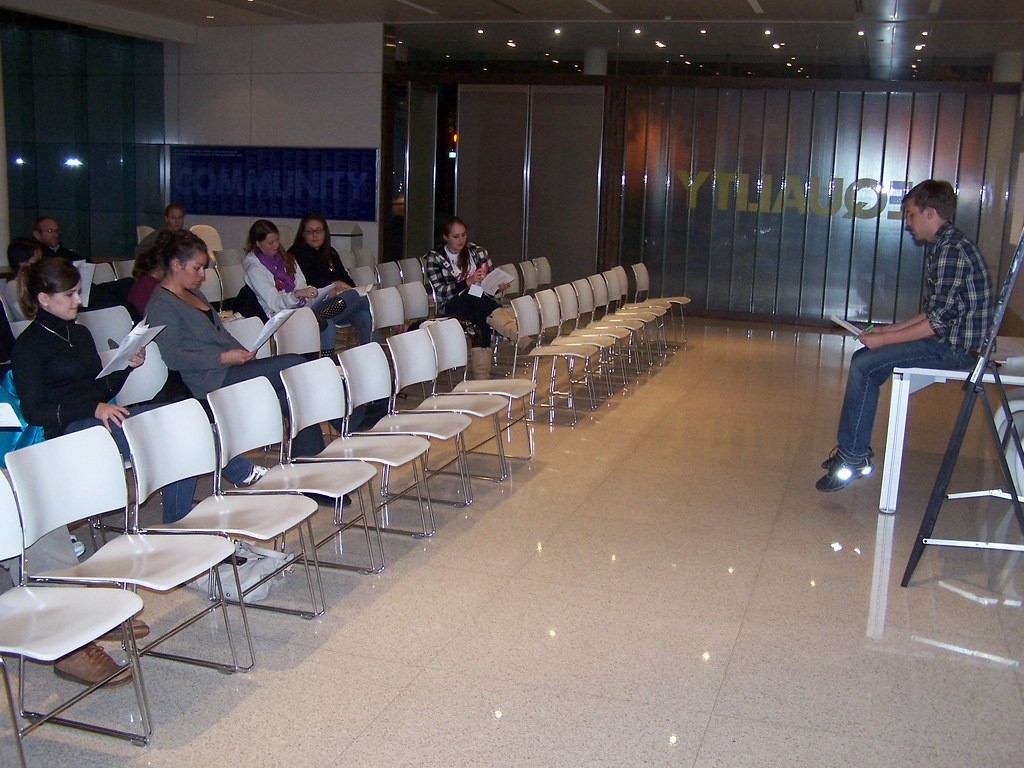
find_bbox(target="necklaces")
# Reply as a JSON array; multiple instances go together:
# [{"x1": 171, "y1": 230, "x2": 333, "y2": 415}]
[{"x1": 36, "y1": 321, "x2": 73, "y2": 347}]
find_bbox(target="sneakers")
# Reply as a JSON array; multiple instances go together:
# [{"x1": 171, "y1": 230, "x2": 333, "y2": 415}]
[
  {"x1": 815, "y1": 456, "x2": 872, "y2": 492},
  {"x1": 821, "y1": 446, "x2": 874, "y2": 470},
  {"x1": 53, "y1": 640, "x2": 132, "y2": 688},
  {"x1": 98, "y1": 620, "x2": 149, "y2": 641}
]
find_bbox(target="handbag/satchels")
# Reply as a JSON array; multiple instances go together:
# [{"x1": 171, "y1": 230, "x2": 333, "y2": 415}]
[{"x1": 185, "y1": 541, "x2": 295, "y2": 603}]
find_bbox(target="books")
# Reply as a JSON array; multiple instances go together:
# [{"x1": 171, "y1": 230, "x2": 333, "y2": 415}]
[
  {"x1": 96, "y1": 314, "x2": 166, "y2": 379},
  {"x1": 337, "y1": 284, "x2": 372, "y2": 295},
  {"x1": 304, "y1": 283, "x2": 337, "y2": 307},
  {"x1": 249, "y1": 310, "x2": 297, "y2": 351},
  {"x1": 474, "y1": 268, "x2": 514, "y2": 297},
  {"x1": 72, "y1": 259, "x2": 96, "y2": 308},
  {"x1": 830, "y1": 314, "x2": 862, "y2": 336}
]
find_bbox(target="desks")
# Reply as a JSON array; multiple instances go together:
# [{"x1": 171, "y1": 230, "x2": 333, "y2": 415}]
[{"x1": 867, "y1": 337, "x2": 1023, "y2": 514}]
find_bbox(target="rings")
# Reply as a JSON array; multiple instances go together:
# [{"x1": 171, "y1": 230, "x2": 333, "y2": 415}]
[{"x1": 311, "y1": 292, "x2": 314, "y2": 296}]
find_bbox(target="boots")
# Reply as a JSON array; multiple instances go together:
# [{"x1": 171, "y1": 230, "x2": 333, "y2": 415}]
[
  {"x1": 486, "y1": 308, "x2": 536, "y2": 354},
  {"x1": 321, "y1": 349, "x2": 334, "y2": 357},
  {"x1": 314, "y1": 296, "x2": 346, "y2": 330},
  {"x1": 471, "y1": 347, "x2": 492, "y2": 380}
]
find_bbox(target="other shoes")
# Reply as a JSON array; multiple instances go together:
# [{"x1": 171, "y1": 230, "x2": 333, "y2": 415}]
[
  {"x1": 358, "y1": 398, "x2": 389, "y2": 431},
  {"x1": 234, "y1": 465, "x2": 272, "y2": 488},
  {"x1": 303, "y1": 492, "x2": 352, "y2": 507}
]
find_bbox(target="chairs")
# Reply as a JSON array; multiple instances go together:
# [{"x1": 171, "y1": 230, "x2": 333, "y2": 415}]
[{"x1": 0, "y1": 225, "x2": 691, "y2": 768}]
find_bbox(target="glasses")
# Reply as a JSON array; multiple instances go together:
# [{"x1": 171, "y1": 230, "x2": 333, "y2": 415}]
[
  {"x1": 302, "y1": 228, "x2": 326, "y2": 234},
  {"x1": 41, "y1": 229, "x2": 58, "y2": 233}
]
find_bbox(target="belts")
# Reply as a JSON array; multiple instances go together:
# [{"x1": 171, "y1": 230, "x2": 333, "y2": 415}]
[{"x1": 969, "y1": 349, "x2": 979, "y2": 357}]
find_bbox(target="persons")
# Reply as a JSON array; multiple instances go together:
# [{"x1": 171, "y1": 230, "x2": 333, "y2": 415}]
[
  {"x1": 130, "y1": 231, "x2": 366, "y2": 506},
  {"x1": 243, "y1": 219, "x2": 361, "y2": 364},
  {"x1": 135, "y1": 205, "x2": 185, "y2": 259},
  {"x1": 816, "y1": 180, "x2": 994, "y2": 492},
  {"x1": 33, "y1": 218, "x2": 79, "y2": 265},
  {"x1": 427, "y1": 217, "x2": 537, "y2": 381},
  {"x1": 13, "y1": 258, "x2": 271, "y2": 523},
  {"x1": 0, "y1": 300, "x2": 16, "y2": 381},
  {"x1": 0, "y1": 471, "x2": 150, "y2": 687},
  {"x1": 285, "y1": 212, "x2": 382, "y2": 346},
  {"x1": 6, "y1": 239, "x2": 141, "y2": 328}
]
